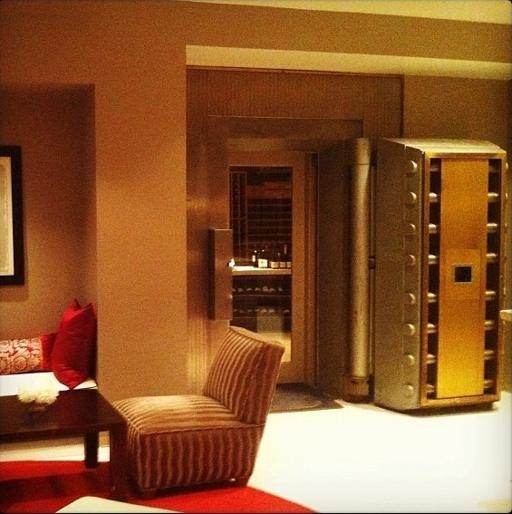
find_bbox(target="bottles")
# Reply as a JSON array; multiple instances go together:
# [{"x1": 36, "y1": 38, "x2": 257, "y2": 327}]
[{"x1": 250, "y1": 243, "x2": 292, "y2": 270}]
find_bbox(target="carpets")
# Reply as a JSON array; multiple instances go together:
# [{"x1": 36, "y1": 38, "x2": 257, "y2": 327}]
[
  {"x1": 270, "y1": 381, "x2": 344, "y2": 413},
  {"x1": 1, "y1": 460, "x2": 318, "y2": 514}
]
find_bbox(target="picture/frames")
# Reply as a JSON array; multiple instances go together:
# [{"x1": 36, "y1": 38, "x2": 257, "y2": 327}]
[{"x1": 0, "y1": 143, "x2": 23, "y2": 288}]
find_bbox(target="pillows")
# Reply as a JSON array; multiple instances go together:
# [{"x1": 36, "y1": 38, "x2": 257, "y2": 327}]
[
  {"x1": 49, "y1": 299, "x2": 98, "y2": 390},
  {"x1": 0, "y1": 333, "x2": 54, "y2": 373}
]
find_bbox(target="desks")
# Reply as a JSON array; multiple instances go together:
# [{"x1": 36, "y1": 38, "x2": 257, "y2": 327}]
[{"x1": 0, "y1": 394, "x2": 128, "y2": 498}]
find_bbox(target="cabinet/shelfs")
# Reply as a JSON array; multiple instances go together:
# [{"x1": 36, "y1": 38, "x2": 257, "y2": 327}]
[{"x1": 230, "y1": 264, "x2": 293, "y2": 320}]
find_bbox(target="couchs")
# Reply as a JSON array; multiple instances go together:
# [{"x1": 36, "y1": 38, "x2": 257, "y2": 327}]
[
  {"x1": 111, "y1": 325, "x2": 287, "y2": 498},
  {"x1": 0, "y1": 335, "x2": 98, "y2": 395}
]
